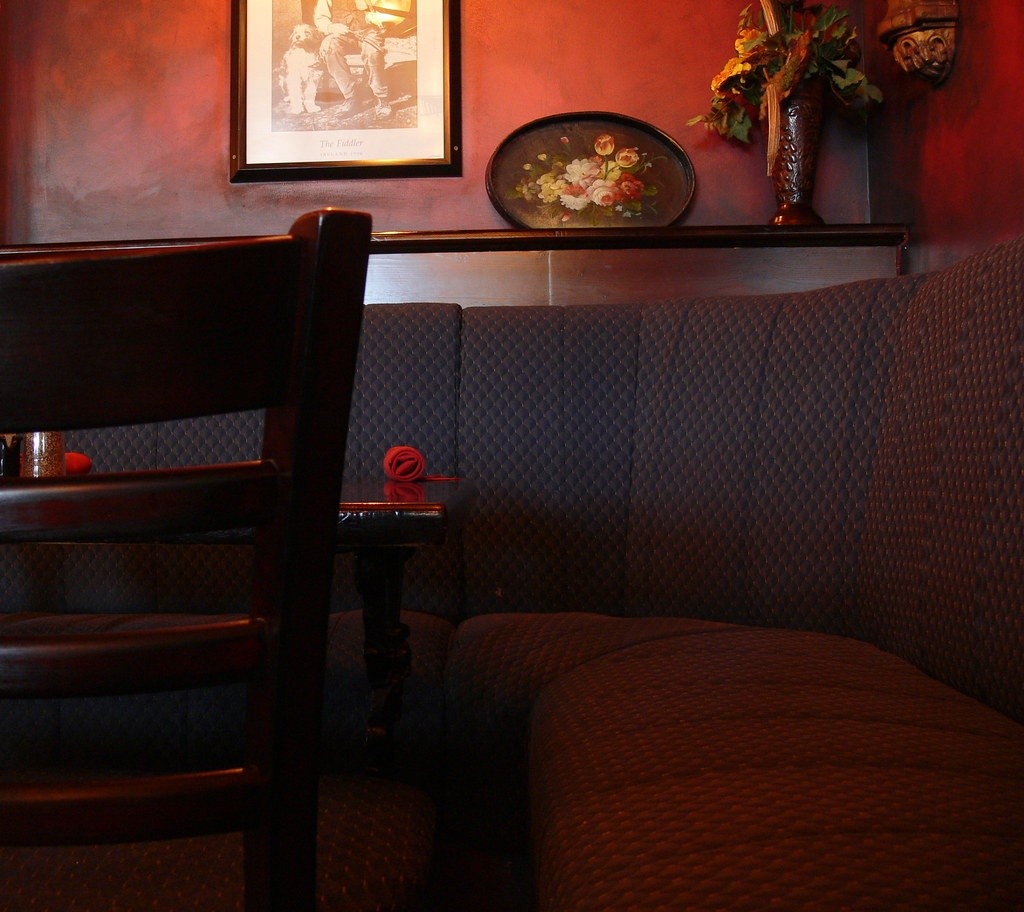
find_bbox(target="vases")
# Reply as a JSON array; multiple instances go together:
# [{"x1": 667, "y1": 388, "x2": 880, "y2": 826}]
[{"x1": 760, "y1": 76, "x2": 825, "y2": 225}]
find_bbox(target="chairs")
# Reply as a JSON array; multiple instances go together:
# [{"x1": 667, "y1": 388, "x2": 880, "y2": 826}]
[{"x1": 0, "y1": 210, "x2": 437, "y2": 912}]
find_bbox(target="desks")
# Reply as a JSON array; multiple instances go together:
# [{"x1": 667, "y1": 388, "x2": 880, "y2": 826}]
[{"x1": 74, "y1": 478, "x2": 475, "y2": 777}]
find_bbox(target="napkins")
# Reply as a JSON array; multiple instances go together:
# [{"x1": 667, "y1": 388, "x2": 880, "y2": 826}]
[
  {"x1": 65, "y1": 451, "x2": 92, "y2": 474},
  {"x1": 383, "y1": 480, "x2": 425, "y2": 501},
  {"x1": 383, "y1": 446, "x2": 462, "y2": 481}
]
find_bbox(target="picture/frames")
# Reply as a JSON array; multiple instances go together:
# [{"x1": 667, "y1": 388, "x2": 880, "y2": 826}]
[{"x1": 229, "y1": 0, "x2": 462, "y2": 222}]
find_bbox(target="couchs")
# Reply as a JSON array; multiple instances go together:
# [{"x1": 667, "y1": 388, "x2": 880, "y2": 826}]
[{"x1": 0, "y1": 234, "x2": 1024, "y2": 912}]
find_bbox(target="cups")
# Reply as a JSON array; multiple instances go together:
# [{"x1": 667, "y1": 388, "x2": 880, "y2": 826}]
[{"x1": 21, "y1": 431, "x2": 66, "y2": 479}]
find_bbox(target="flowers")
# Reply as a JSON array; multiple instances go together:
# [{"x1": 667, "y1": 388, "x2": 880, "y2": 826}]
[{"x1": 686, "y1": 0, "x2": 883, "y2": 144}]
